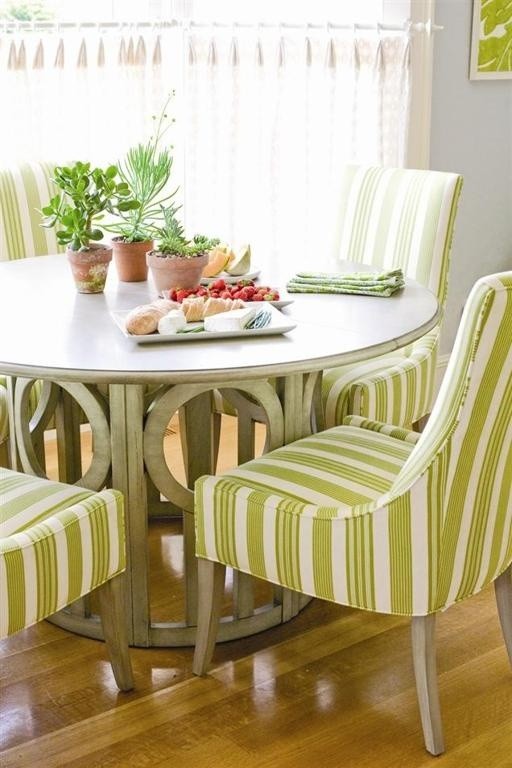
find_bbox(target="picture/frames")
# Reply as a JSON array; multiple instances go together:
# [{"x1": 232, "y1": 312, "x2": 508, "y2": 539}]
[{"x1": 467, "y1": 0, "x2": 512, "y2": 80}]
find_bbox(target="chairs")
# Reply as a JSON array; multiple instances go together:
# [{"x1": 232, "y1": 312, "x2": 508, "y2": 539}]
[
  {"x1": 0, "y1": 166, "x2": 182, "y2": 470},
  {"x1": 210, "y1": 163, "x2": 464, "y2": 477},
  {"x1": 0, "y1": 465, "x2": 135, "y2": 693},
  {"x1": 191, "y1": 270, "x2": 512, "y2": 755}
]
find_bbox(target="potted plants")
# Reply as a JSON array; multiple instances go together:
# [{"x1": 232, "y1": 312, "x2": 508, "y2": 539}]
[
  {"x1": 145, "y1": 204, "x2": 221, "y2": 300},
  {"x1": 33, "y1": 162, "x2": 140, "y2": 294},
  {"x1": 95, "y1": 88, "x2": 184, "y2": 282}
]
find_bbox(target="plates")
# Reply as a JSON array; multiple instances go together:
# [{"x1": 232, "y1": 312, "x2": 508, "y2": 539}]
[{"x1": 107, "y1": 268, "x2": 295, "y2": 344}]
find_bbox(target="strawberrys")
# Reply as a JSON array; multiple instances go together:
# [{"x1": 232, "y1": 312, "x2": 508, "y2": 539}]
[{"x1": 168, "y1": 278, "x2": 280, "y2": 304}]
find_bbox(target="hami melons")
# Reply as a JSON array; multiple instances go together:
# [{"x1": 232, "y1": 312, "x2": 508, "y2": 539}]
[{"x1": 202, "y1": 243, "x2": 251, "y2": 278}]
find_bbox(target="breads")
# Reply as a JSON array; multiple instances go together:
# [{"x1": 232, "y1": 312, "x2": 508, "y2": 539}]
[
  {"x1": 125, "y1": 299, "x2": 179, "y2": 335},
  {"x1": 182, "y1": 297, "x2": 246, "y2": 323}
]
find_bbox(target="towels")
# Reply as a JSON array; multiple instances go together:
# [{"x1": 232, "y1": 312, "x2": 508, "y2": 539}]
[{"x1": 286, "y1": 270, "x2": 405, "y2": 297}]
